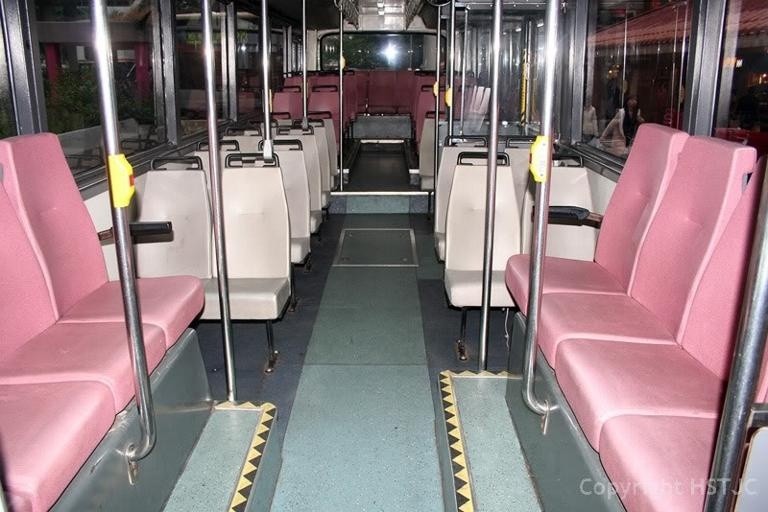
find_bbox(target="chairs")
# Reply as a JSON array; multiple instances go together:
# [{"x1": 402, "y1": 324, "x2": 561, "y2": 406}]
[{"x1": 0, "y1": 66, "x2": 766, "y2": 512}]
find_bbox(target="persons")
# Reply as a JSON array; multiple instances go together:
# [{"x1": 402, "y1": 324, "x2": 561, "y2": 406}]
[
  {"x1": 730, "y1": 86, "x2": 767, "y2": 131},
  {"x1": 599, "y1": 97, "x2": 645, "y2": 159},
  {"x1": 582, "y1": 96, "x2": 599, "y2": 144}
]
